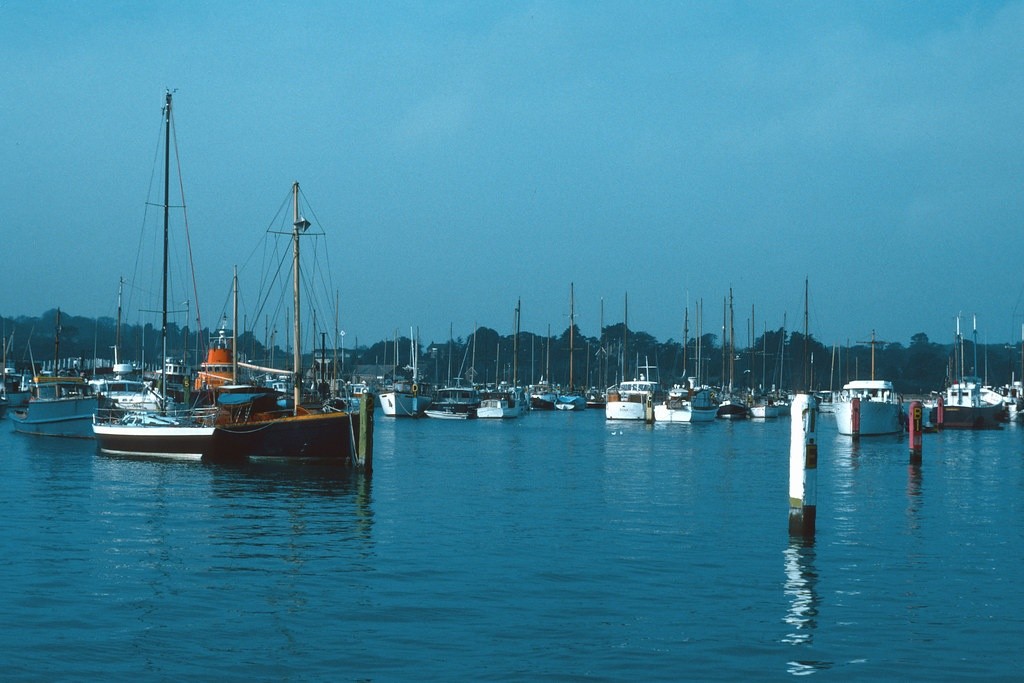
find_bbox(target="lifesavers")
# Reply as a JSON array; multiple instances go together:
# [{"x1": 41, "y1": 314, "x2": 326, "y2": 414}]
[
  {"x1": 361, "y1": 388, "x2": 368, "y2": 394},
  {"x1": 412, "y1": 384, "x2": 419, "y2": 391}
]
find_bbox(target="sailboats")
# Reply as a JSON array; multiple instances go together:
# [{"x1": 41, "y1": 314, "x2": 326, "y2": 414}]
[{"x1": 0, "y1": 84, "x2": 1024, "y2": 470}]
